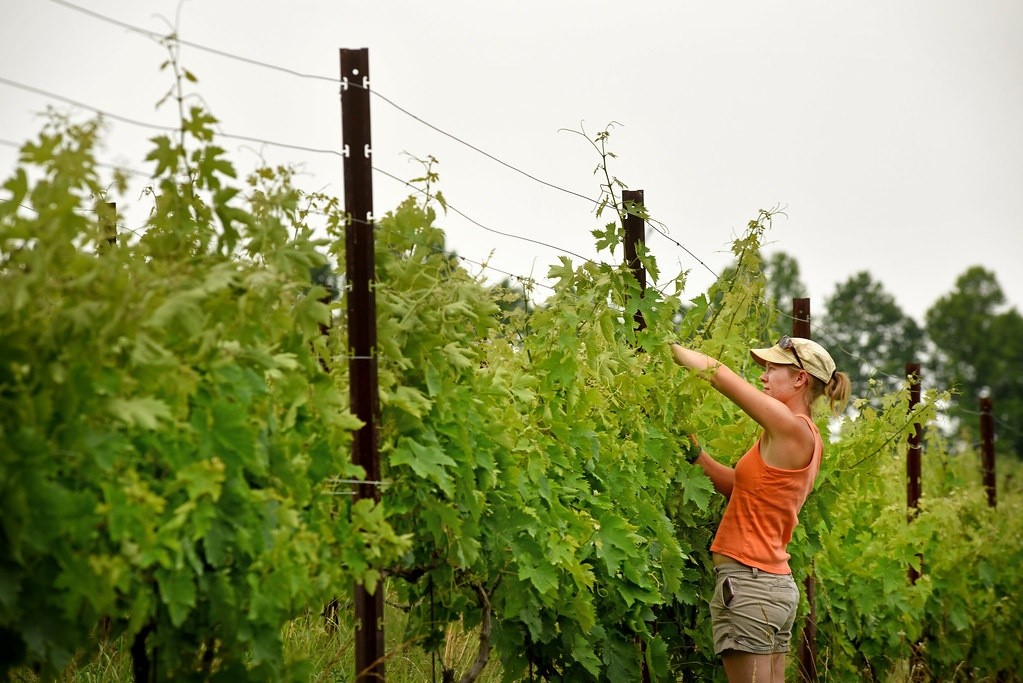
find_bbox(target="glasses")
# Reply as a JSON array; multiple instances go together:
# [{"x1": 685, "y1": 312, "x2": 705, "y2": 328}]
[{"x1": 776, "y1": 334, "x2": 810, "y2": 386}]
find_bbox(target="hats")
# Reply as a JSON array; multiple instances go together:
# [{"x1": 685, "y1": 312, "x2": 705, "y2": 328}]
[{"x1": 750, "y1": 337, "x2": 836, "y2": 386}]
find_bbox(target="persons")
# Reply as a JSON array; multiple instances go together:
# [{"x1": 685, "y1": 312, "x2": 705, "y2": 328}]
[{"x1": 672, "y1": 338, "x2": 851, "y2": 683}]
